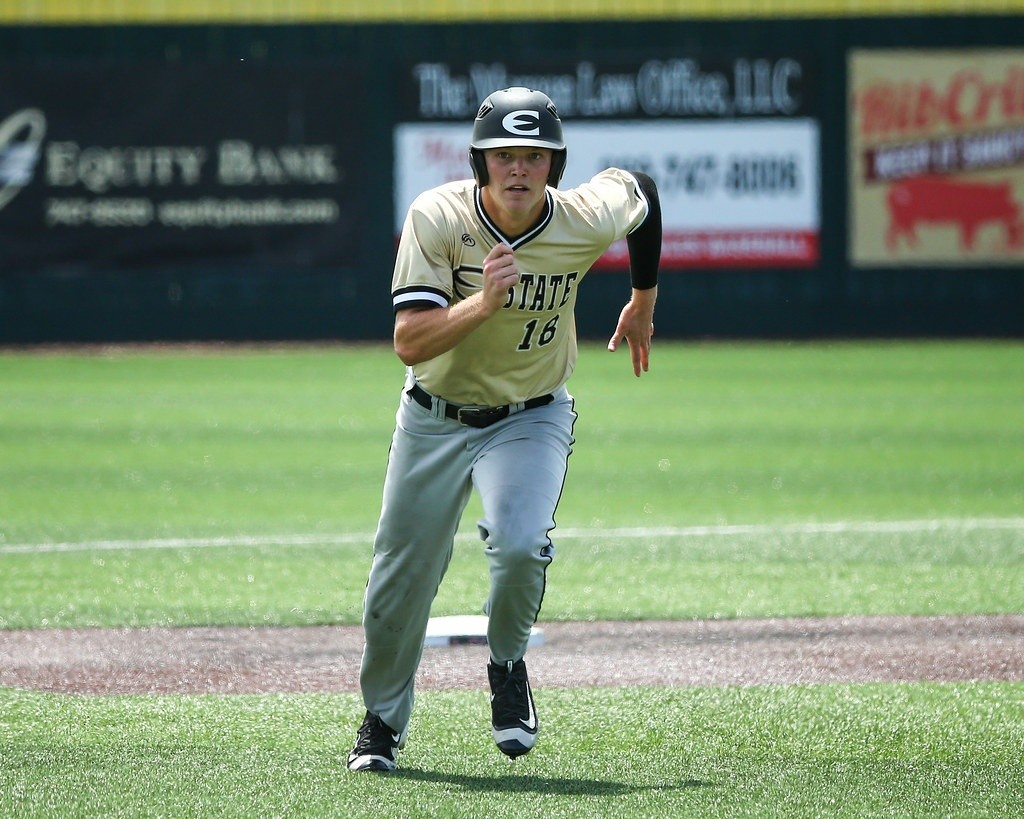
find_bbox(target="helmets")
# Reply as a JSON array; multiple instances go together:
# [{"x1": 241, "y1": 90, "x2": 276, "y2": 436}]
[{"x1": 468, "y1": 87, "x2": 567, "y2": 189}]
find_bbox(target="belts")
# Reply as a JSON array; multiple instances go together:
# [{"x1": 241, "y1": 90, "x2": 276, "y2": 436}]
[{"x1": 409, "y1": 384, "x2": 552, "y2": 428}]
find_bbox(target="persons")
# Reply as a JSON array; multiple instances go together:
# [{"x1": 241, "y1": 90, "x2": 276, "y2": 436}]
[{"x1": 347, "y1": 87, "x2": 662, "y2": 768}]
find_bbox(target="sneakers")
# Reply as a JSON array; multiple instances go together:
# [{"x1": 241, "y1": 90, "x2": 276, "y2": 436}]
[
  {"x1": 486, "y1": 655, "x2": 538, "y2": 756},
  {"x1": 347, "y1": 710, "x2": 408, "y2": 773}
]
